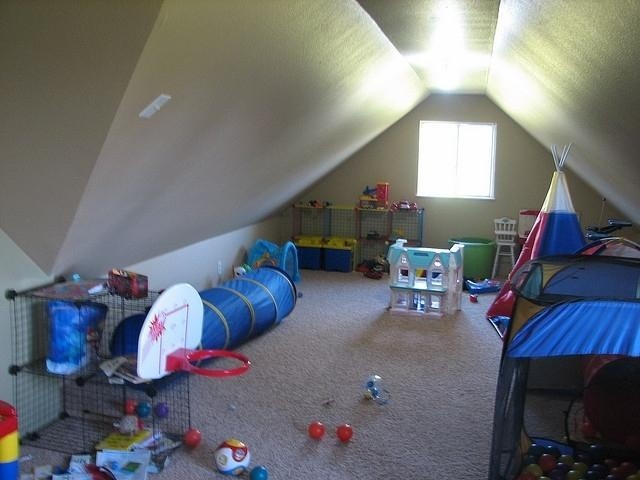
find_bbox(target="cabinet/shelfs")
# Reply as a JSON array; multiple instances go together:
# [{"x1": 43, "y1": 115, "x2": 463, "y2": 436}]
[
  {"x1": 291, "y1": 201, "x2": 424, "y2": 274},
  {"x1": 5, "y1": 275, "x2": 191, "y2": 474}
]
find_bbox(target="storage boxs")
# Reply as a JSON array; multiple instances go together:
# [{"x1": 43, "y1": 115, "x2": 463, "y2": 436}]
[{"x1": 296, "y1": 246, "x2": 352, "y2": 273}]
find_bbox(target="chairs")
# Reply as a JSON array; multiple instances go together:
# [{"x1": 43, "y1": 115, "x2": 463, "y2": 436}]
[{"x1": 491, "y1": 215, "x2": 517, "y2": 280}]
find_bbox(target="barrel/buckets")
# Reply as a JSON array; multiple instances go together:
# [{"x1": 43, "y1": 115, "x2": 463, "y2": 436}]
[
  {"x1": 517, "y1": 209, "x2": 540, "y2": 243},
  {"x1": 448, "y1": 237, "x2": 497, "y2": 279}
]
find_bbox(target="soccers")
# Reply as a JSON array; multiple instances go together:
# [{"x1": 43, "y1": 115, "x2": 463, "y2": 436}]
[{"x1": 215, "y1": 439, "x2": 251, "y2": 476}]
